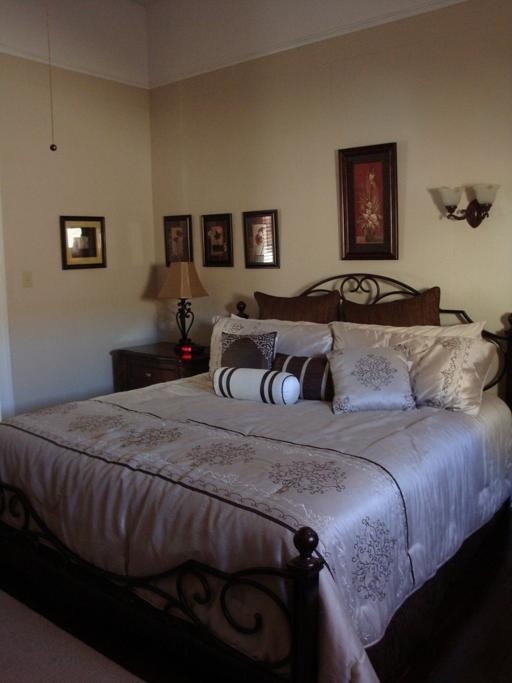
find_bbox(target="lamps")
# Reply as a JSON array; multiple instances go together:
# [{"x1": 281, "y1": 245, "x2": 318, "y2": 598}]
[
  {"x1": 155, "y1": 260, "x2": 210, "y2": 354},
  {"x1": 438, "y1": 181, "x2": 501, "y2": 228}
]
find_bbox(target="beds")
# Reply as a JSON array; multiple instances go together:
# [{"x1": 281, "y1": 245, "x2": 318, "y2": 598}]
[{"x1": 0, "y1": 272, "x2": 512, "y2": 682}]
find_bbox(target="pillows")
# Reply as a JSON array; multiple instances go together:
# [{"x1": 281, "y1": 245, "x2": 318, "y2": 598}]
[{"x1": 206, "y1": 284, "x2": 499, "y2": 420}]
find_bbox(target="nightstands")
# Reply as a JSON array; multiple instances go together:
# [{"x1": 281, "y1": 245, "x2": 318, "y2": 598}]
[{"x1": 108, "y1": 340, "x2": 209, "y2": 393}]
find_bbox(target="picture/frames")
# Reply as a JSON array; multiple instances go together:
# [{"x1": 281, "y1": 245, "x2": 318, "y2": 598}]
[
  {"x1": 57, "y1": 213, "x2": 108, "y2": 270},
  {"x1": 160, "y1": 213, "x2": 193, "y2": 267},
  {"x1": 337, "y1": 140, "x2": 398, "y2": 259},
  {"x1": 242, "y1": 208, "x2": 280, "y2": 270},
  {"x1": 201, "y1": 211, "x2": 235, "y2": 267}
]
